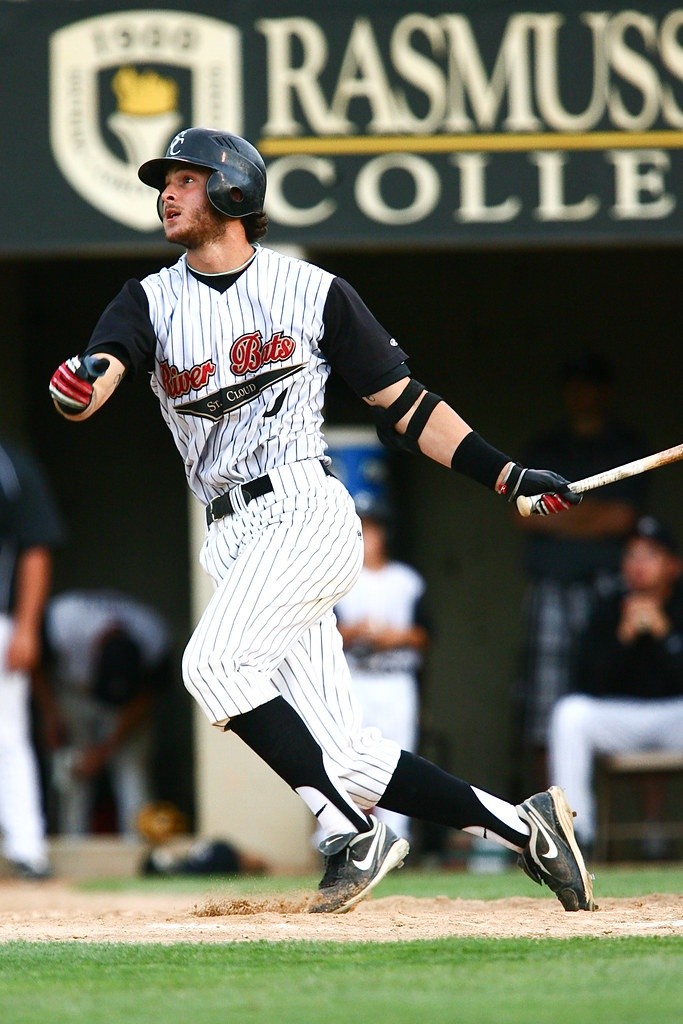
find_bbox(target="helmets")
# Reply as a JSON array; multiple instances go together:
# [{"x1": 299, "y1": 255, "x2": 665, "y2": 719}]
[
  {"x1": 354, "y1": 490, "x2": 390, "y2": 525},
  {"x1": 137, "y1": 128, "x2": 266, "y2": 224}
]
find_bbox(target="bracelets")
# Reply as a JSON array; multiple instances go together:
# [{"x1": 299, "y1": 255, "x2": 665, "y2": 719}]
[{"x1": 451, "y1": 430, "x2": 510, "y2": 493}]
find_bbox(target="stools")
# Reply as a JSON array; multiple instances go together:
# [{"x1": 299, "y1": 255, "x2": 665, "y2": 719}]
[{"x1": 594, "y1": 772, "x2": 683, "y2": 860}]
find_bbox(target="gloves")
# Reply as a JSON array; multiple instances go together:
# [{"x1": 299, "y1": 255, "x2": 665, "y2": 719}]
[
  {"x1": 49, "y1": 354, "x2": 110, "y2": 410},
  {"x1": 498, "y1": 463, "x2": 583, "y2": 516}
]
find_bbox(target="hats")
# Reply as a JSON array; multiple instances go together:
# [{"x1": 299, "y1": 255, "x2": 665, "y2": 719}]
[{"x1": 629, "y1": 516, "x2": 677, "y2": 554}]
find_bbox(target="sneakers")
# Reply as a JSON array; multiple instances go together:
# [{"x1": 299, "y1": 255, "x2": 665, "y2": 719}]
[
  {"x1": 309, "y1": 814, "x2": 409, "y2": 915},
  {"x1": 510, "y1": 785, "x2": 598, "y2": 913}
]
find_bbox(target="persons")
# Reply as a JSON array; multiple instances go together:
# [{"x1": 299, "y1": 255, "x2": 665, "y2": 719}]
[
  {"x1": 333, "y1": 517, "x2": 433, "y2": 837},
  {"x1": 49, "y1": 127, "x2": 599, "y2": 913},
  {"x1": 40, "y1": 592, "x2": 168, "y2": 837},
  {"x1": 0, "y1": 483, "x2": 61, "y2": 881},
  {"x1": 548, "y1": 514, "x2": 683, "y2": 846},
  {"x1": 509, "y1": 350, "x2": 672, "y2": 862}
]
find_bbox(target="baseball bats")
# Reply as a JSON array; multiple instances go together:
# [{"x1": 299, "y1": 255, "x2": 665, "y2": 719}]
[{"x1": 516, "y1": 444, "x2": 683, "y2": 518}]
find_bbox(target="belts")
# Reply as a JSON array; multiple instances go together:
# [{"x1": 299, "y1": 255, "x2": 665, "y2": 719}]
[{"x1": 206, "y1": 459, "x2": 337, "y2": 525}]
